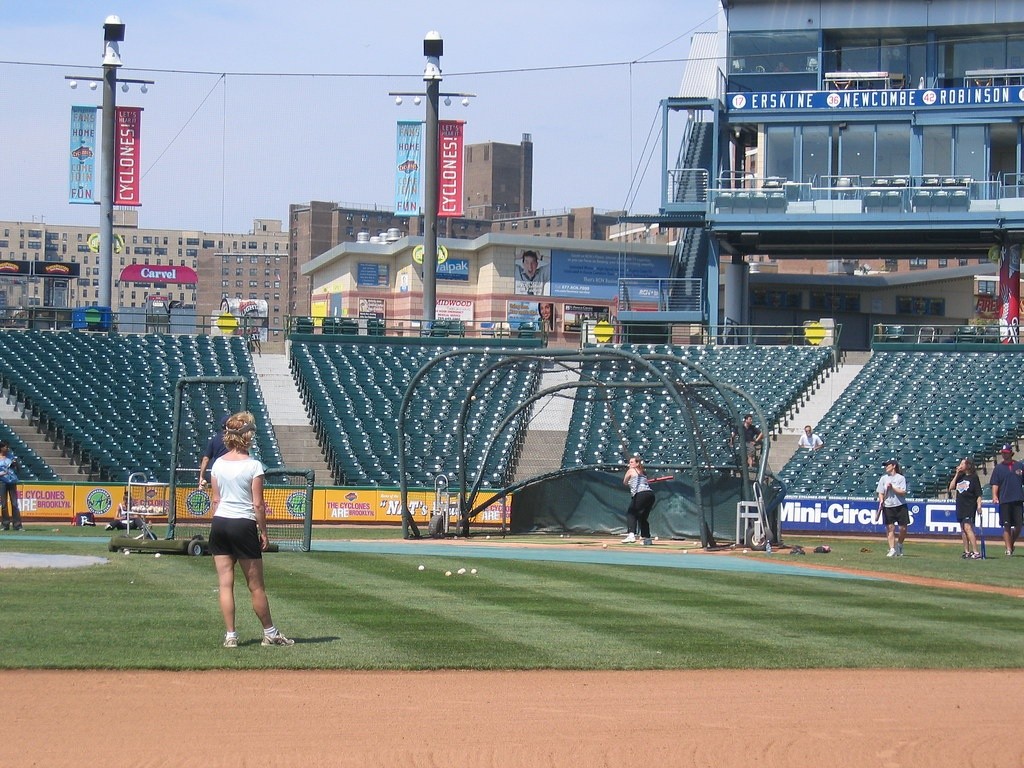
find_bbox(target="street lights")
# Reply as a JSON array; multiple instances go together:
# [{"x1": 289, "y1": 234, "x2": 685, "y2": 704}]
[
  {"x1": 389, "y1": 30, "x2": 477, "y2": 337},
  {"x1": 65, "y1": 14, "x2": 155, "y2": 307}
]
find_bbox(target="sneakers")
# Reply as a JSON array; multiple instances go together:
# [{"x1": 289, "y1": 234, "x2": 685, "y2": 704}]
[
  {"x1": 962, "y1": 551, "x2": 982, "y2": 561},
  {"x1": 1005, "y1": 546, "x2": 1014, "y2": 556},
  {"x1": 640, "y1": 540, "x2": 652, "y2": 545},
  {"x1": 223, "y1": 634, "x2": 238, "y2": 648},
  {"x1": 887, "y1": 542, "x2": 903, "y2": 557},
  {"x1": 261, "y1": 630, "x2": 294, "y2": 648},
  {"x1": 621, "y1": 536, "x2": 635, "y2": 543}
]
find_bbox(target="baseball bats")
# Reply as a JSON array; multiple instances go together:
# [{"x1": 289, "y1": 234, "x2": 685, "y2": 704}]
[
  {"x1": 979, "y1": 513, "x2": 986, "y2": 559},
  {"x1": 874, "y1": 483, "x2": 890, "y2": 522},
  {"x1": 647, "y1": 475, "x2": 674, "y2": 483}
]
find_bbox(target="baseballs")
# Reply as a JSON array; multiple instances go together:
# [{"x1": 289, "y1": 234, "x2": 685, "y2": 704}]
[
  {"x1": 445, "y1": 571, "x2": 451, "y2": 576},
  {"x1": 684, "y1": 550, "x2": 688, "y2": 553},
  {"x1": 199, "y1": 479, "x2": 208, "y2": 491},
  {"x1": 470, "y1": 568, "x2": 478, "y2": 575},
  {"x1": 560, "y1": 534, "x2": 564, "y2": 538},
  {"x1": 125, "y1": 549, "x2": 130, "y2": 555},
  {"x1": 486, "y1": 535, "x2": 491, "y2": 540},
  {"x1": 602, "y1": 544, "x2": 608, "y2": 549},
  {"x1": 654, "y1": 537, "x2": 658, "y2": 541},
  {"x1": 694, "y1": 542, "x2": 698, "y2": 547},
  {"x1": 155, "y1": 553, "x2": 161, "y2": 559},
  {"x1": 418, "y1": 565, "x2": 425, "y2": 571},
  {"x1": 131, "y1": 505, "x2": 167, "y2": 513},
  {"x1": 743, "y1": 549, "x2": 747, "y2": 553},
  {"x1": 457, "y1": 567, "x2": 467, "y2": 575}
]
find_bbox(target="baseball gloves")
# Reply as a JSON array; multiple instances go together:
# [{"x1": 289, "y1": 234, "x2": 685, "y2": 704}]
[{"x1": 859, "y1": 546, "x2": 874, "y2": 554}]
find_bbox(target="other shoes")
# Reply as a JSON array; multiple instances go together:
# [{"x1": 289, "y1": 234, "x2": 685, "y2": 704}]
[
  {"x1": 0, "y1": 528, "x2": 9, "y2": 530},
  {"x1": 18, "y1": 528, "x2": 25, "y2": 531}
]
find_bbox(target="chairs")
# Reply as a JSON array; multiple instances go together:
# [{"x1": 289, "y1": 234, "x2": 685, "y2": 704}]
[
  {"x1": 713, "y1": 174, "x2": 979, "y2": 213},
  {"x1": 0, "y1": 316, "x2": 1024, "y2": 500}
]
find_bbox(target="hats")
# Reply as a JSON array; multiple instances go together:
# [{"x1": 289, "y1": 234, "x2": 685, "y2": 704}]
[
  {"x1": 1001, "y1": 444, "x2": 1013, "y2": 453},
  {"x1": 881, "y1": 460, "x2": 897, "y2": 466},
  {"x1": 220, "y1": 416, "x2": 230, "y2": 429}
]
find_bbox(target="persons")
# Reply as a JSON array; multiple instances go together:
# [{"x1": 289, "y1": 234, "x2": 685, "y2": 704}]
[
  {"x1": 208, "y1": 410, "x2": 295, "y2": 648},
  {"x1": 538, "y1": 303, "x2": 553, "y2": 330},
  {"x1": 947, "y1": 457, "x2": 983, "y2": 559},
  {"x1": 515, "y1": 249, "x2": 550, "y2": 296},
  {"x1": 990, "y1": 445, "x2": 1024, "y2": 556},
  {"x1": 105, "y1": 491, "x2": 152, "y2": 531},
  {"x1": 199, "y1": 417, "x2": 249, "y2": 492},
  {"x1": 622, "y1": 457, "x2": 655, "y2": 545},
  {"x1": 876, "y1": 460, "x2": 910, "y2": 556},
  {"x1": 730, "y1": 414, "x2": 763, "y2": 467},
  {"x1": 0, "y1": 439, "x2": 26, "y2": 532},
  {"x1": 798, "y1": 426, "x2": 824, "y2": 451}
]
